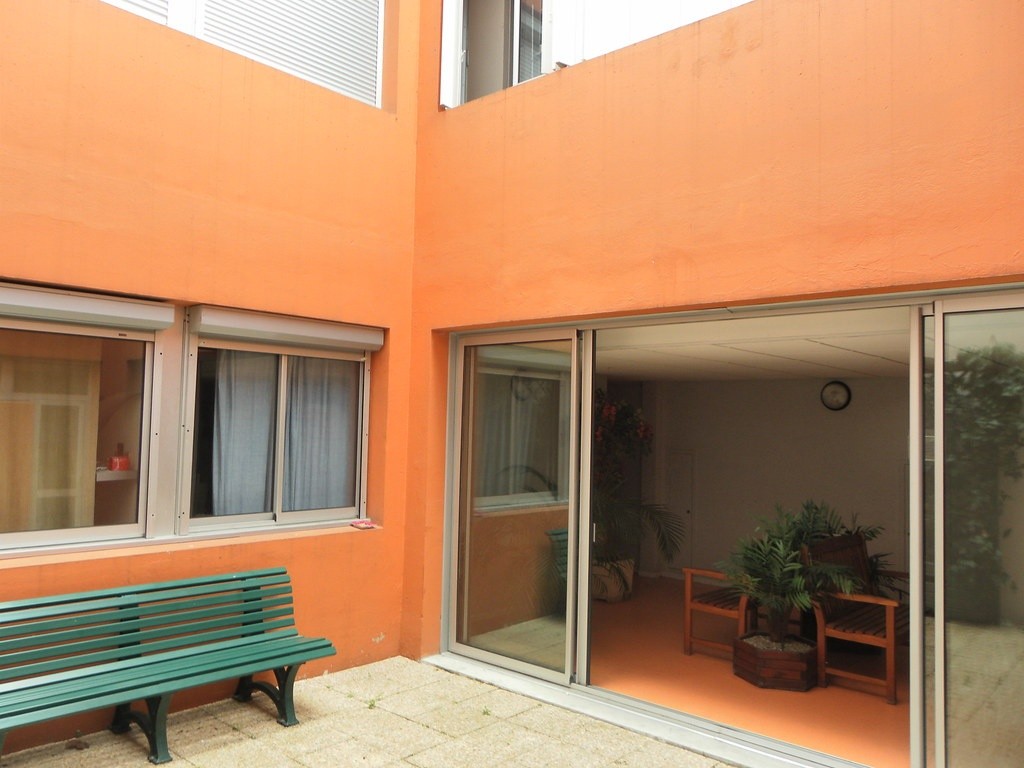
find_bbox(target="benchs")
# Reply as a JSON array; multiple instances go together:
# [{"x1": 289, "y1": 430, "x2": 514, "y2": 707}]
[
  {"x1": 681, "y1": 566, "x2": 757, "y2": 656},
  {"x1": 0, "y1": 566, "x2": 336, "y2": 768},
  {"x1": 803, "y1": 531, "x2": 911, "y2": 704},
  {"x1": 545, "y1": 528, "x2": 567, "y2": 610}
]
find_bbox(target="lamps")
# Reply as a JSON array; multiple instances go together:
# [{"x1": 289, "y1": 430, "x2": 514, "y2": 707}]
[{"x1": 820, "y1": 381, "x2": 851, "y2": 410}]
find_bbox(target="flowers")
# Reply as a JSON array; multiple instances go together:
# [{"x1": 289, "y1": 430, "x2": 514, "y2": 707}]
[{"x1": 561, "y1": 389, "x2": 684, "y2": 602}]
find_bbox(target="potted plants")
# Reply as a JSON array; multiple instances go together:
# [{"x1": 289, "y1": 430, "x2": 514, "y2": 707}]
[{"x1": 714, "y1": 499, "x2": 909, "y2": 691}]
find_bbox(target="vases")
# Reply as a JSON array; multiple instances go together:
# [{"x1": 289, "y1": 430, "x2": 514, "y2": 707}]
[{"x1": 589, "y1": 542, "x2": 640, "y2": 601}]
